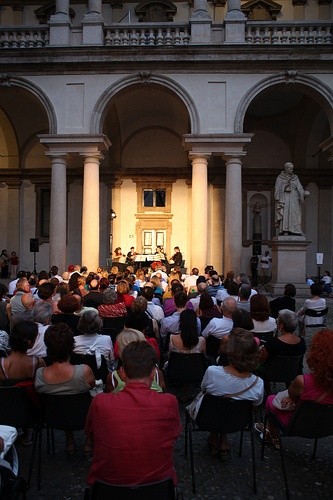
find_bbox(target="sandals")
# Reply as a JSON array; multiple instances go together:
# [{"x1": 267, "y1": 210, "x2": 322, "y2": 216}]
[
  {"x1": 208, "y1": 440, "x2": 218, "y2": 453},
  {"x1": 254, "y1": 423, "x2": 271, "y2": 434},
  {"x1": 220, "y1": 447, "x2": 230, "y2": 459},
  {"x1": 259, "y1": 432, "x2": 280, "y2": 449}
]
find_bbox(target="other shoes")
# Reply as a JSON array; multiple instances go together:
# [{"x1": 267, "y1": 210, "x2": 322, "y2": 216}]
[
  {"x1": 84, "y1": 444, "x2": 92, "y2": 457},
  {"x1": 21, "y1": 431, "x2": 34, "y2": 446},
  {"x1": 64, "y1": 440, "x2": 75, "y2": 453}
]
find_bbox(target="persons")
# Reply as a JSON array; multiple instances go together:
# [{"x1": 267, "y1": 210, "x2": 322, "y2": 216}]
[
  {"x1": 106, "y1": 327, "x2": 165, "y2": 393},
  {"x1": 306, "y1": 271, "x2": 331, "y2": 286},
  {"x1": 166, "y1": 247, "x2": 182, "y2": 274},
  {"x1": 250, "y1": 251, "x2": 272, "y2": 285},
  {"x1": 155, "y1": 246, "x2": 163, "y2": 254},
  {"x1": 274, "y1": 162, "x2": 310, "y2": 236},
  {"x1": 269, "y1": 284, "x2": 296, "y2": 318},
  {"x1": 184, "y1": 327, "x2": 264, "y2": 460},
  {"x1": 296, "y1": 283, "x2": 326, "y2": 337},
  {"x1": 0, "y1": 320, "x2": 48, "y2": 445},
  {"x1": 257, "y1": 309, "x2": 307, "y2": 395},
  {"x1": 36, "y1": 323, "x2": 95, "y2": 456},
  {"x1": 0, "y1": 265, "x2": 277, "y2": 366},
  {"x1": 0, "y1": 250, "x2": 19, "y2": 278},
  {"x1": 255, "y1": 328, "x2": 333, "y2": 449},
  {"x1": 114, "y1": 247, "x2": 138, "y2": 264},
  {"x1": 81, "y1": 340, "x2": 189, "y2": 496}
]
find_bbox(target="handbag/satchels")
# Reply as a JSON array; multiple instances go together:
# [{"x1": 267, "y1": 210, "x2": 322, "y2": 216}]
[
  {"x1": 0, "y1": 424, "x2": 18, "y2": 476},
  {"x1": 185, "y1": 392, "x2": 204, "y2": 420},
  {"x1": 272, "y1": 389, "x2": 296, "y2": 410}
]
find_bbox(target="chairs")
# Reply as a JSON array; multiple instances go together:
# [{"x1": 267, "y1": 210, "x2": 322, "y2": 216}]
[{"x1": 0, "y1": 306, "x2": 333, "y2": 500}]
